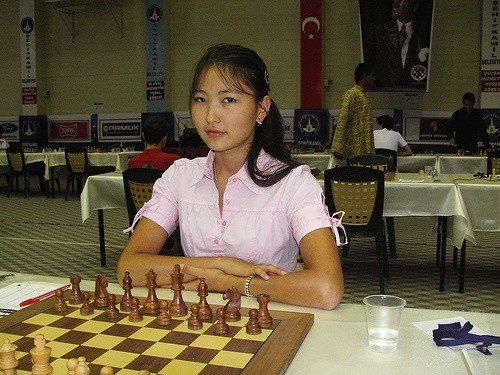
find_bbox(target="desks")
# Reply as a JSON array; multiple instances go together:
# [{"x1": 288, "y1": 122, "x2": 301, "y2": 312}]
[{"x1": 0, "y1": 150, "x2": 500, "y2": 375}]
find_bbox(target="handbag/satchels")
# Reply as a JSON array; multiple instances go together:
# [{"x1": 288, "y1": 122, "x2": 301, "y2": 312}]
[{"x1": 326, "y1": 154, "x2": 341, "y2": 169}]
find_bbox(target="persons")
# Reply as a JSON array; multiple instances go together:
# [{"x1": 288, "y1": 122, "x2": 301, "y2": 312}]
[
  {"x1": 372, "y1": 115, "x2": 412, "y2": 156},
  {"x1": 364, "y1": 0, "x2": 426, "y2": 87},
  {"x1": 128, "y1": 114, "x2": 181, "y2": 171},
  {"x1": 332, "y1": 62, "x2": 377, "y2": 165},
  {"x1": 439, "y1": 93, "x2": 490, "y2": 155},
  {"x1": 117, "y1": 45, "x2": 344, "y2": 311}
]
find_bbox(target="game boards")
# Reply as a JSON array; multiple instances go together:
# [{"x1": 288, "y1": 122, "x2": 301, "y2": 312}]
[{"x1": 0, "y1": 286, "x2": 316, "y2": 375}]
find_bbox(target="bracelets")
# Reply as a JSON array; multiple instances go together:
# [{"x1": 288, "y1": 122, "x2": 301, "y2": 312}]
[{"x1": 244, "y1": 275, "x2": 255, "y2": 297}]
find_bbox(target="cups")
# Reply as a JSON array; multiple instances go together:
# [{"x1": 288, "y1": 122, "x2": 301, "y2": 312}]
[
  {"x1": 423, "y1": 166, "x2": 437, "y2": 183},
  {"x1": 363, "y1": 295, "x2": 406, "y2": 352}
]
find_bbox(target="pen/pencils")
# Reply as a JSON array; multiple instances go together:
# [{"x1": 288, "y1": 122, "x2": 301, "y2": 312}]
[{"x1": 19, "y1": 284, "x2": 71, "y2": 306}]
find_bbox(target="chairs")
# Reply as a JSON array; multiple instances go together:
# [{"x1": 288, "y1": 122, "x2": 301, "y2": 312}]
[
  {"x1": 5, "y1": 146, "x2": 61, "y2": 198},
  {"x1": 64, "y1": 148, "x2": 95, "y2": 203},
  {"x1": 323, "y1": 148, "x2": 397, "y2": 294},
  {"x1": 123, "y1": 168, "x2": 183, "y2": 258}
]
